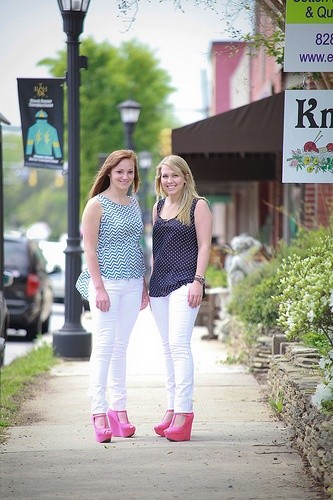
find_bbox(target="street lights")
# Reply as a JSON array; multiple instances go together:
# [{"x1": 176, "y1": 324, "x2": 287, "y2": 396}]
[
  {"x1": 52, "y1": 0, "x2": 92, "y2": 361},
  {"x1": 117, "y1": 97, "x2": 142, "y2": 151}
]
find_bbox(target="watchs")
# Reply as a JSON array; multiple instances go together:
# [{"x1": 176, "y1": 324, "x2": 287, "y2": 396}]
[{"x1": 194, "y1": 277, "x2": 205, "y2": 284}]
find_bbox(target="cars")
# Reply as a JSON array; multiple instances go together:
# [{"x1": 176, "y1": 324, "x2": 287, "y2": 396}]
[{"x1": 1, "y1": 232, "x2": 88, "y2": 366}]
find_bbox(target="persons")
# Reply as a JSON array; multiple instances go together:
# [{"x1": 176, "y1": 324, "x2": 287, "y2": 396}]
[
  {"x1": 82, "y1": 149, "x2": 150, "y2": 443},
  {"x1": 148, "y1": 155, "x2": 213, "y2": 442}
]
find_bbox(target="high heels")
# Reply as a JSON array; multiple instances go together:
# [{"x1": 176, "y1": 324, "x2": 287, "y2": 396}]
[
  {"x1": 107, "y1": 408, "x2": 135, "y2": 438},
  {"x1": 154, "y1": 410, "x2": 194, "y2": 441},
  {"x1": 91, "y1": 413, "x2": 112, "y2": 443}
]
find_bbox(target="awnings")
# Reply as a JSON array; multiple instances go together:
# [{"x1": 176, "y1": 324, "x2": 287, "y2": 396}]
[{"x1": 173, "y1": 84, "x2": 304, "y2": 181}]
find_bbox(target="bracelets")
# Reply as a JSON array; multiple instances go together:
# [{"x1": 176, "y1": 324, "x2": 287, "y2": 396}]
[{"x1": 194, "y1": 274, "x2": 204, "y2": 278}]
